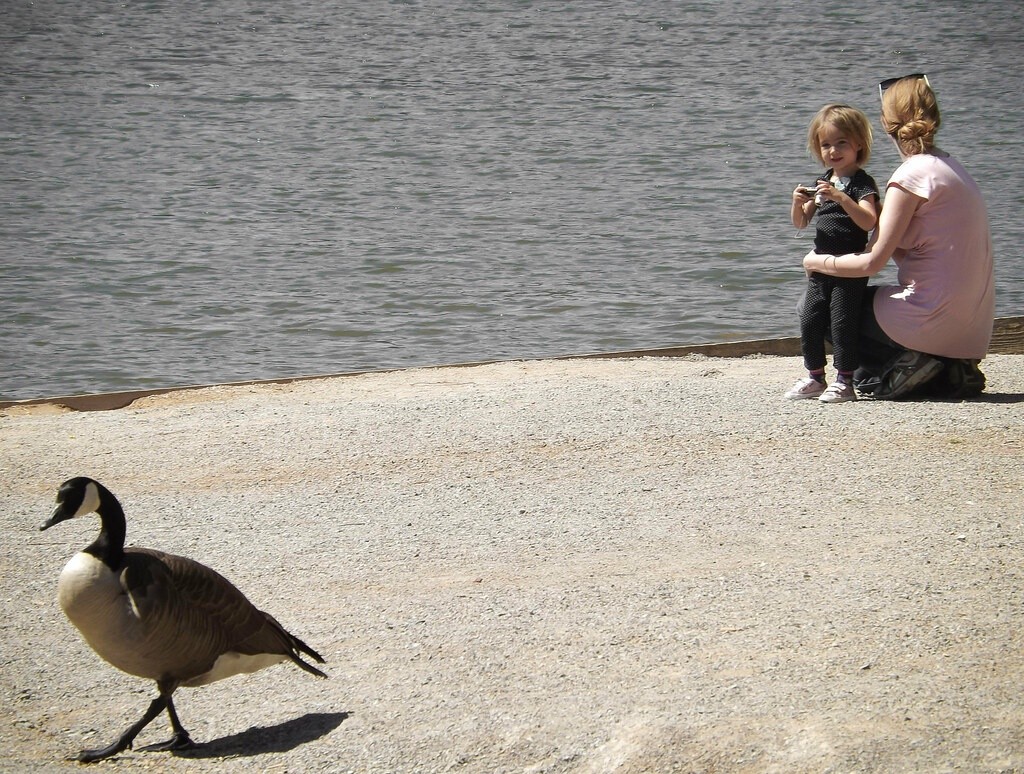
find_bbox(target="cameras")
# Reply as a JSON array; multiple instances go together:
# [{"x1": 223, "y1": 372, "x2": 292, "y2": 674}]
[{"x1": 804, "y1": 187, "x2": 828, "y2": 207}]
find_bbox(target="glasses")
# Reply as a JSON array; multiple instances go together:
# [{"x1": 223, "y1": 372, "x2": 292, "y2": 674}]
[{"x1": 878, "y1": 72, "x2": 931, "y2": 108}]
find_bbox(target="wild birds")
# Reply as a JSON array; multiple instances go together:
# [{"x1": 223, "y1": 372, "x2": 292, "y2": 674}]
[{"x1": 37, "y1": 476, "x2": 330, "y2": 765}]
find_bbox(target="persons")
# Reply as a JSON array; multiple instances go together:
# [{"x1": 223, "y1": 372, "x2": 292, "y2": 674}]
[
  {"x1": 801, "y1": 73, "x2": 994, "y2": 403},
  {"x1": 783, "y1": 104, "x2": 877, "y2": 404}
]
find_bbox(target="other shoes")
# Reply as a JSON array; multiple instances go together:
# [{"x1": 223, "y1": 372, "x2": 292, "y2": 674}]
[
  {"x1": 870, "y1": 350, "x2": 986, "y2": 400},
  {"x1": 783, "y1": 377, "x2": 857, "y2": 403}
]
